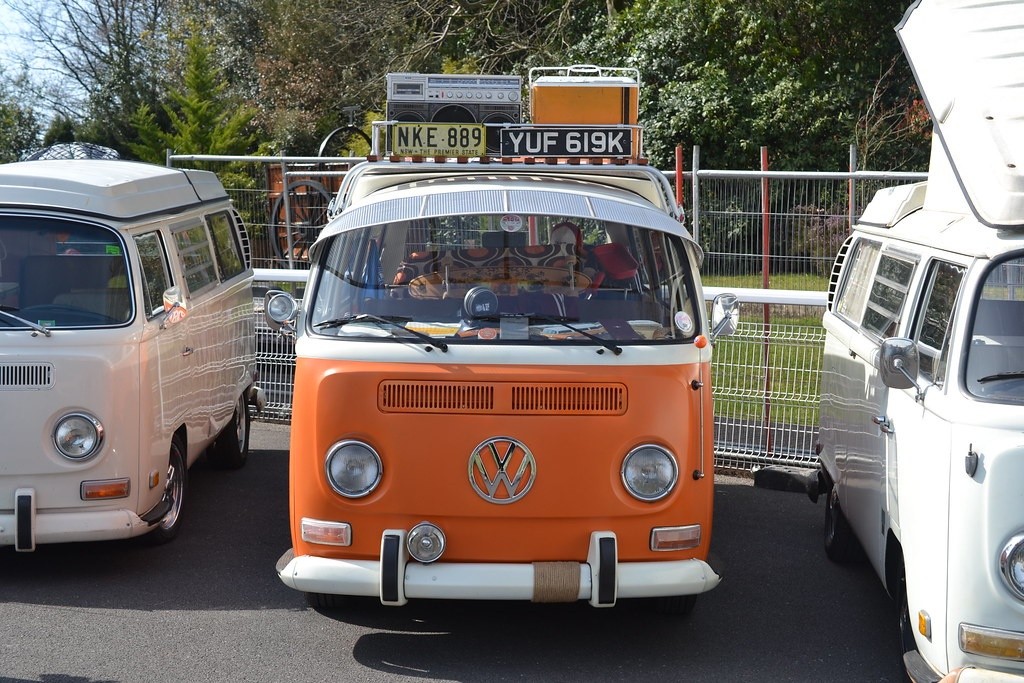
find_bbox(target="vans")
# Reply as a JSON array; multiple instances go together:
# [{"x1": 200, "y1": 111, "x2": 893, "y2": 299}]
[
  {"x1": 0, "y1": 158, "x2": 267, "y2": 553},
  {"x1": 266, "y1": 65, "x2": 742, "y2": 621},
  {"x1": 812, "y1": 2, "x2": 1023, "y2": 683}
]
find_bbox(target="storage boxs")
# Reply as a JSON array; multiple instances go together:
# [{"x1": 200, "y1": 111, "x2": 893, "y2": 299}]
[{"x1": 529, "y1": 64, "x2": 640, "y2": 158}]
